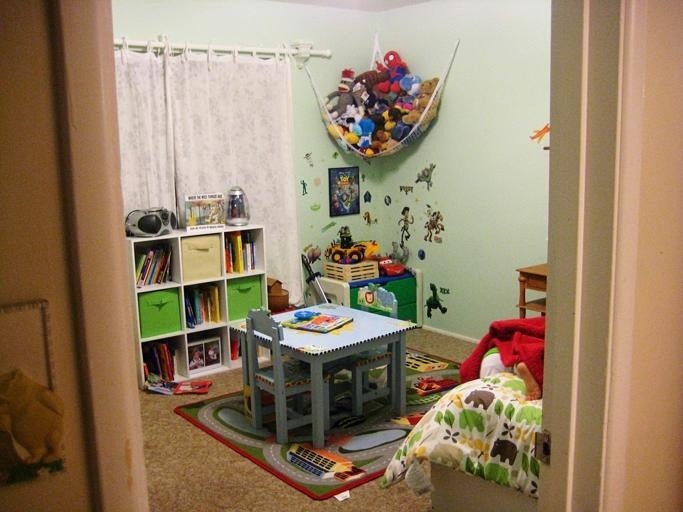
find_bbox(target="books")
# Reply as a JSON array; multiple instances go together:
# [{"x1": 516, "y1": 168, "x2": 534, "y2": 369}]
[
  {"x1": 283, "y1": 309, "x2": 354, "y2": 333},
  {"x1": 129, "y1": 231, "x2": 264, "y2": 381}
]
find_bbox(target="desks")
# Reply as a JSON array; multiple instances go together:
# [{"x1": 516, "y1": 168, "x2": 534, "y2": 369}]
[
  {"x1": 230, "y1": 303, "x2": 419, "y2": 449},
  {"x1": 516, "y1": 263, "x2": 547, "y2": 319}
]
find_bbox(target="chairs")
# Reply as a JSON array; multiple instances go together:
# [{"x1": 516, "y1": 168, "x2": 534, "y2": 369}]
[
  {"x1": 246, "y1": 307, "x2": 332, "y2": 444},
  {"x1": 329, "y1": 282, "x2": 398, "y2": 416}
]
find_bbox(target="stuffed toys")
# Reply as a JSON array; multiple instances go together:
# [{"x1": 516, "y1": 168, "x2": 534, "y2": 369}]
[{"x1": 322, "y1": 51, "x2": 441, "y2": 156}]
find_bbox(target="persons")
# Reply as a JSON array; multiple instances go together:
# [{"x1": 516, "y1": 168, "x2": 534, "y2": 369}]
[{"x1": 481, "y1": 345, "x2": 541, "y2": 401}]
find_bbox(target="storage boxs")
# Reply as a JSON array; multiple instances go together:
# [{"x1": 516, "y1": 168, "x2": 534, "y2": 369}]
[
  {"x1": 227, "y1": 275, "x2": 262, "y2": 322},
  {"x1": 181, "y1": 235, "x2": 223, "y2": 282},
  {"x1": 139, "y1": 289, "x2": 181, "y2": 337}
]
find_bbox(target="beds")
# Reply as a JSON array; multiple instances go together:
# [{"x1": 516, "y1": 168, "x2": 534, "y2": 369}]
[{"x1": 377, "y1": 373, "x2": 544, "y2": 512}]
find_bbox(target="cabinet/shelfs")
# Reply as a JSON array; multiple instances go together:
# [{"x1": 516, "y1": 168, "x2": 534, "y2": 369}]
[
  {"x1": 126, "y1": 224, "x2": 268, "y2": 391},
  {"x1": 308, "y1": 268, "x2": 423, "y2": 329}
]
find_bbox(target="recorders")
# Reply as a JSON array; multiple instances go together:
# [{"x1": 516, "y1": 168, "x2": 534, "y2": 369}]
[{"x1": 125, "y1": 206, "x2": 178, "y2": 237}]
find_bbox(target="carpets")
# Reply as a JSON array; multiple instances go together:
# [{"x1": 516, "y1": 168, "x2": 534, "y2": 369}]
[{"x1": 174, "y1": 346, "x2": 463, "y2": 500}]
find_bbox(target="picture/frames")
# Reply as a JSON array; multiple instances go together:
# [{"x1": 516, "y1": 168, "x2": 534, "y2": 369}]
[{"x1": 329, "y1": 167, "x2": 360, "y2": 217}]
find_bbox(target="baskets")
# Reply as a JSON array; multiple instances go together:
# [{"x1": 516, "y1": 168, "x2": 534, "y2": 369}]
[{"x1": 323, "y1": 259, "x2": 380, "y2": 283}]
